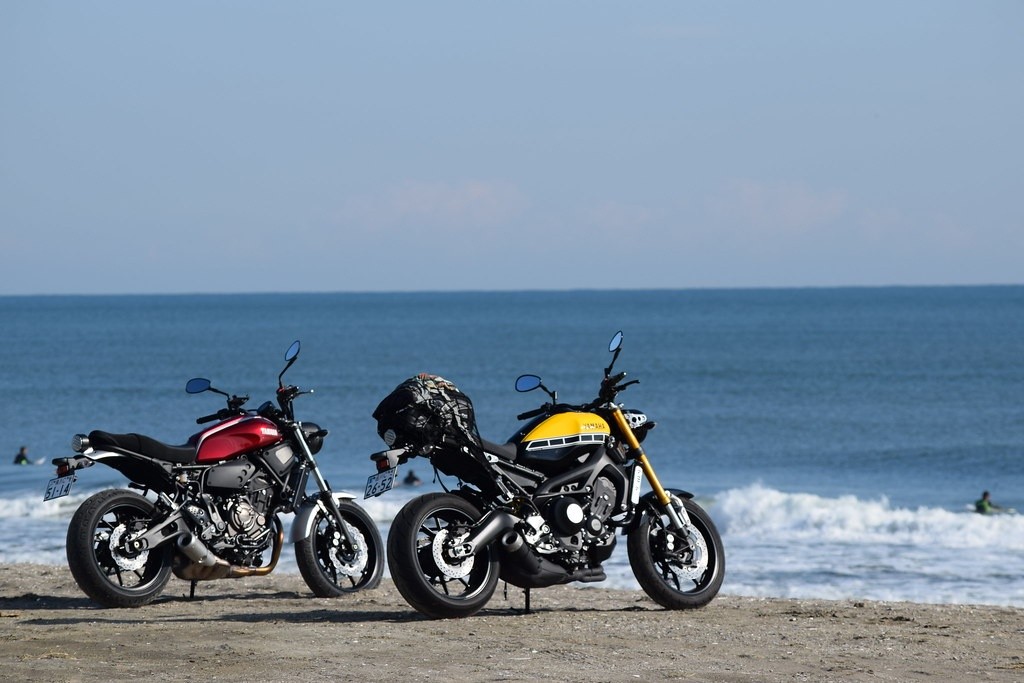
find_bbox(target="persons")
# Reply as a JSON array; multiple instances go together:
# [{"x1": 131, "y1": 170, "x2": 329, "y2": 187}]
[
  {"x1": 973, "y1": 489, "x2": 1001, "y2": 515},
  {"x1": 12, "y1": 446, "x2": 34, "y2": 465},
  {"x1": 403, "y1": 470, "x2": 422, "y2": 486}
]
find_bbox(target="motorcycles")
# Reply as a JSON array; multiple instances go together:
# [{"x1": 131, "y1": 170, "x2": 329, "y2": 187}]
[
  {"x1": 43, "y1": 340, "x2": 385, "y2": 608},
  {"x1": 364, "y1": 331, "x2": 725, "y2": 617}
]
